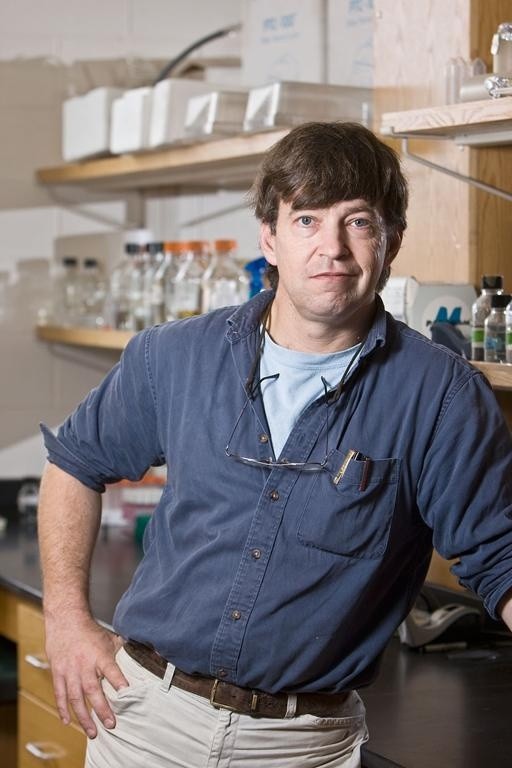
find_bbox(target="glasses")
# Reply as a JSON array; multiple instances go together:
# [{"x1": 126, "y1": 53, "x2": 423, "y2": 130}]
[{"x1": 222, "y1": 371, "x2": 335, "y2": 474}]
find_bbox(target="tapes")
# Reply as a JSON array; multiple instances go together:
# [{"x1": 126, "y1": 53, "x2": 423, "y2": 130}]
[
  {"x1": 430, "y1": 608, "x2": 448, "y2": 625},
  {"x1": 454, "y1": 324, "x2": 472, "y2": 340}
]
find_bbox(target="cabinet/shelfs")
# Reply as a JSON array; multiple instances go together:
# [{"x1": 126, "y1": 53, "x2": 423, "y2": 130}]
[
  {"x1": 380, "y1": 95, "x2": 512, "y2": 205},
  {"x1": 34, "y1": 96, "x2": 291, "y2": 231},
  {"x1": 468, "y1": 361, "x2": 512, "y2": 390},
  {"x1": 36, "y1": 324, "x2": 139, "y2": 373}
]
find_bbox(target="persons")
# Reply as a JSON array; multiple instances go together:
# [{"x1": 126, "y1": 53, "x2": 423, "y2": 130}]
[{"x1": 37, "y1": 120, "x2": 510, "y2": 767}]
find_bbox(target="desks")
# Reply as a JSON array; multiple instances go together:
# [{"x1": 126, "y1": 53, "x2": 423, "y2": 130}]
[{"x1": 1, "y1": 516, "x2": 512, "y2": 767}]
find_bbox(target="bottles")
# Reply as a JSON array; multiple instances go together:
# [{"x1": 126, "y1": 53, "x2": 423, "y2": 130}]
[
  {"x1": 108, "y1": 239, "x2": 251, "y2": 333},
  {"x1": 51, "y1": 254, "x2": 107, "y2": 328},
  {"x1": 470, "y1": 272, "x2": 512, "y2": 364}
]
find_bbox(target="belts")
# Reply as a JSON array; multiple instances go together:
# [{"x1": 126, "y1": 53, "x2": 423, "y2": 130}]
[{"x1": 120, "y1": 635, "x2": 355, "y2": 721}]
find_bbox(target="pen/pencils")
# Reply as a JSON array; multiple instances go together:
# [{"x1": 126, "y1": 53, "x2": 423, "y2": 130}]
[
  {"x1": 333, "y1": 448, "x2": 371, "y2": 492},
  {"x1": 419, "y1": 641, "x2": 468, "y2": 654}
]
